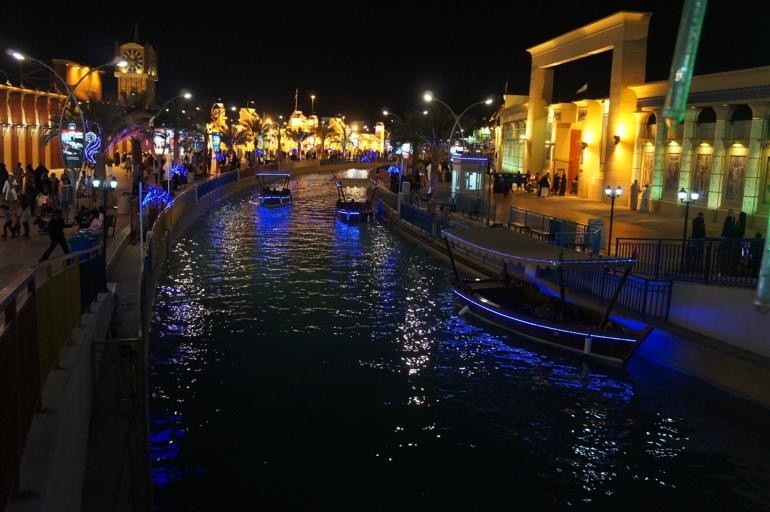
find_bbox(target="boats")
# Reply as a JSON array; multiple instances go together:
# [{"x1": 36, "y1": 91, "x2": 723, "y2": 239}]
[
  {"x1": 437, "y1": 223, "x2": 657, "y2": 368},
  {"x1": 331, "y1": 168, "x2": 382, "y2": 226},
  {"x1": 255, "y1": 172, "x2": 294, "y2": 209}
]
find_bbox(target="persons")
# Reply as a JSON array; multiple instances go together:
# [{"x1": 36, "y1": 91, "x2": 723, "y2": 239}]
[
  {"x1": 206, "y1": 147, "x2": 316, "y2": 170},
  {"x1": 630, "y1": 180, "x2": 650, "y2": 212},
  {"x1": 112, "y1": 149, "x2": 165, "y2": 183},
  {"x1": 2, "y1": 162, "x2": 104, "y2": 263},
  {"x1": 492, "y1": 170, "x2": 583, "y2": 197},
  {"x1": 691, "y1": 206, "x2": 765, "y2": 276}
]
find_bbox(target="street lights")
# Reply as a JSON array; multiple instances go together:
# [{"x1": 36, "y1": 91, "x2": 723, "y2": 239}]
[
  {"x1": 604, "y1": 185, "x2": 623, "y2": 257},
  {"x1": 91, "y1": 174, "x2": 119, "y2": 295},
  {"x1": 310, "y1": 95, "x2": 316, "y2": 112},
  {"x1": 7, "y1": 46, "x2": 130, "y2": 217},
  {"x1": 381, "y1": 89, "x2": 494, "y2": 180},
  {"x1": 677, "y1": 186, "x2": 702, "y2": 274},
  {"x1": 335, "y1": 114, "x2": 347, "y2": 152},
  {"x1": 145, "y1": 88, "x2": 256, "y2": 196}
]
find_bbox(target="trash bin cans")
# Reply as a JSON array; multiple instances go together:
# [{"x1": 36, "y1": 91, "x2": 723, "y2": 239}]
[
  {"x1": 589, "y1": 218, "x2": 605, "y2": 250},
  {"x1": 117, "y1": 196, "x2": 130, "y2": 215},
  {"x1": 401, "y1": 182, "x2": 410, "y2": 193}
]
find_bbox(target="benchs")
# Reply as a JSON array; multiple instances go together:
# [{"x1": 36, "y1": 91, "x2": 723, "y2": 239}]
[
  {"x1": 508, "y1": 222, "x2": 529, "y2": 238},
  {"x1": 531, "y1": 229, "x2": 550, "y2": 244}
]
